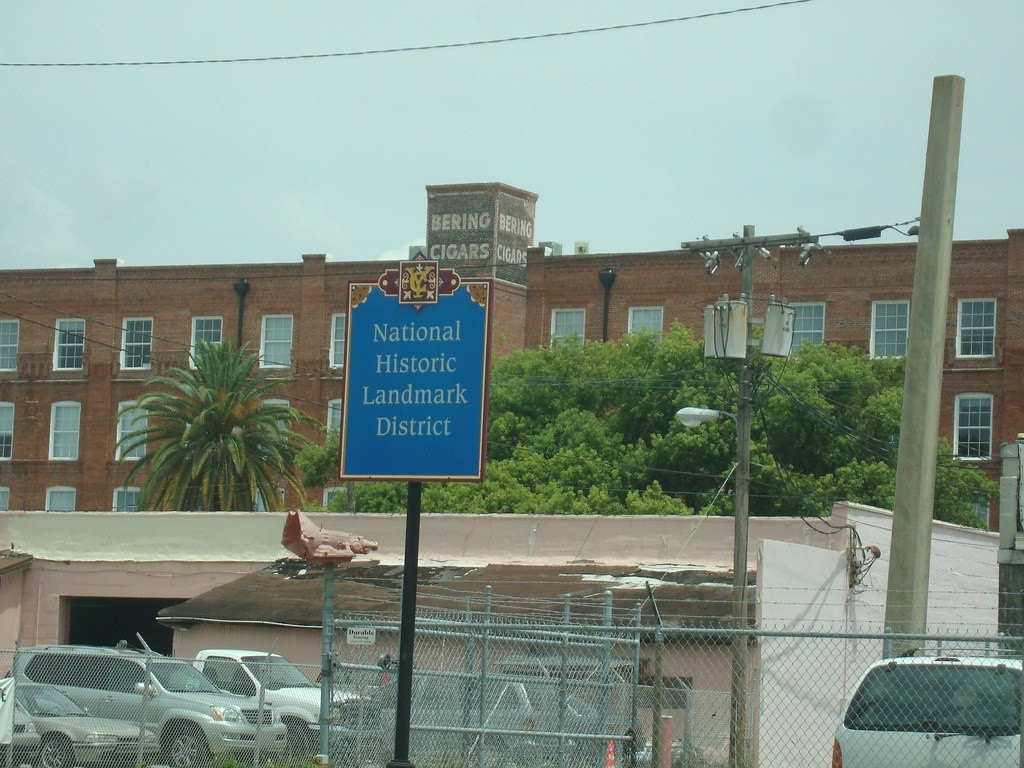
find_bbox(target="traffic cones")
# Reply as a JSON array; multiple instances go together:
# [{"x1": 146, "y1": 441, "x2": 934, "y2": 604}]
[{"x1": 605, "y1": 739, "x2": 615, "y2": 767}]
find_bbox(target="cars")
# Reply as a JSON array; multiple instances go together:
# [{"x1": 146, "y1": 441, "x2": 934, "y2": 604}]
[{"x1": 5, "y1": 677, "x2": 160, "y2": 767}]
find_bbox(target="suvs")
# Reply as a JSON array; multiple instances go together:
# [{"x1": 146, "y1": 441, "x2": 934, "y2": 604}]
[
  {"x1": 4, "y1": 644, "x2": 288, "y2": 767},
  {"x1": 831, "y1": 644, "x2": 1021, "y2": 768},
  {"x1": 336, "y1": 672, "x2": 600, "y2": 768}
]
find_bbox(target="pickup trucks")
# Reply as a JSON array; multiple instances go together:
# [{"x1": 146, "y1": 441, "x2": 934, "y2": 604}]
[{"x1": 194, "y1": 649, "x2": 375, "y2": 761}]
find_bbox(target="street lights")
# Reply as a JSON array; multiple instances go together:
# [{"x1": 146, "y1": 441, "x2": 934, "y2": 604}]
[{"x1": 674, "y1": 410, "x2": 748, "y2": 768}]
